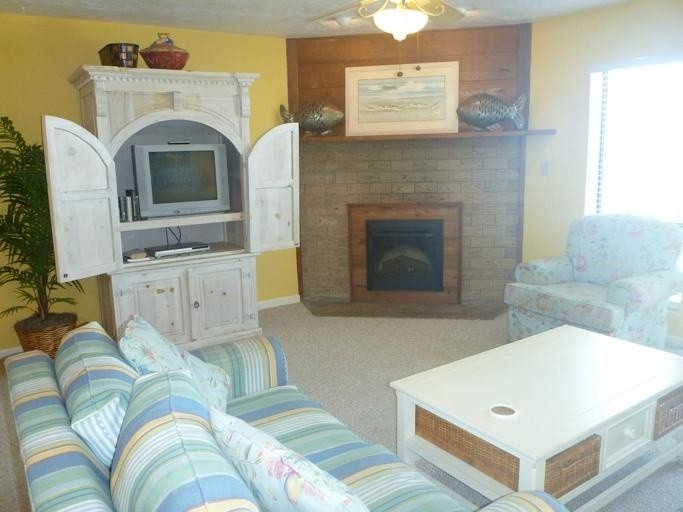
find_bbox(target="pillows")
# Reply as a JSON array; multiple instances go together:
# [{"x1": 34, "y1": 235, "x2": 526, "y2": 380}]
[
  {"x1": 207, "y1": 406, "x2": 365, "y2": 510},
  {"x1": 120, "y1": 314, "x2": 233, "y2": 416}
]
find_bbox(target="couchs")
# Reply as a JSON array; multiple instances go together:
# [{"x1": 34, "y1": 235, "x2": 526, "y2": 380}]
[{"x1": 1, "y1": 319, "x2": 572, "y2": 511}]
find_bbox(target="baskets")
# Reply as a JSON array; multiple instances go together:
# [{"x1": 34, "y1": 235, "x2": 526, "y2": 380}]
[
  {"x1": 15, "y1": 312, "x2": 77, "y2": 359},
  {"x1": 139, "y1": 51, "x2": 190, "y2": 69}
]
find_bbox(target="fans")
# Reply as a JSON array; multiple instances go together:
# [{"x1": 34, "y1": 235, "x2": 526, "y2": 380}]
[{"x1": 304, "y1": 0, "x2": 466, "y2": 26}]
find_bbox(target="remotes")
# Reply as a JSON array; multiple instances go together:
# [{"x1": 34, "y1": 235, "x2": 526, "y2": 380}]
[{"x1": 168, "y1": 141, "x2": 190, "y2": 144}]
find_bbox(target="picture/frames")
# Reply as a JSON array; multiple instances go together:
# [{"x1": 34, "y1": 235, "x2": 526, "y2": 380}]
[{"x1": 344, "y1": 61, "x2": 460, "y2": 137}]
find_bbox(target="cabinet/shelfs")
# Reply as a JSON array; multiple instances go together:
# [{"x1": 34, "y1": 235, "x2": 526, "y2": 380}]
[{"x1": 38, "y1": 64, "x2": 303, "y2": 351}]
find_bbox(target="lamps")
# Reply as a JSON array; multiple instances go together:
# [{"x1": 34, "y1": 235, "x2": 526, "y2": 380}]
[{"x1": 358, "y1": 0, "x2": 445, "y2": 42}]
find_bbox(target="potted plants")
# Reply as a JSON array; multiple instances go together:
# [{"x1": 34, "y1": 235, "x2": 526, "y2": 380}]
[{"x1": 0, "y1": 116, "x2": 86, "y2": 358}]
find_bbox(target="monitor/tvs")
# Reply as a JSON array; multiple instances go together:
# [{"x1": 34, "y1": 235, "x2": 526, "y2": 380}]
[{"x1": 131, "y1": 143, "x2": 231, "y2": 218}]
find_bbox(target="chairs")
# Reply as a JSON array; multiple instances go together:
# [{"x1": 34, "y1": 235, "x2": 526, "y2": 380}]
[{"x1": 504, "y1": 215, "x2": 683, "y2": 341}]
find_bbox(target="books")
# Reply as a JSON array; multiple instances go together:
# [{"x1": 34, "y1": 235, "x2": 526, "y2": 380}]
[
  {"x1": 123, "y1": 248, "x2": 155, "y2": 264},
  {"x1": 119, "y1": 189, "x2": 140, "y2": 223}
]
